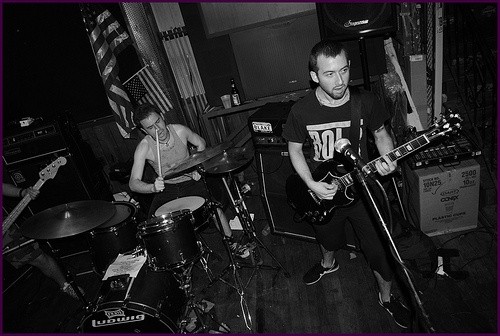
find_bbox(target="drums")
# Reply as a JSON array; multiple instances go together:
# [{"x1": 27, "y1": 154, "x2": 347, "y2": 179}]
[
  {"x1": 152, "y1": 195, "x2": 212, "y2": 231},
  {"x1": 90, "y1": 201, "x2": 138, "y2": 279},
  {"x1": 138, "y1": 209, "x2": 204, "y2": 273},
  {"x1": 78, "y1": 262, "x2": 189, "y2": 334}
]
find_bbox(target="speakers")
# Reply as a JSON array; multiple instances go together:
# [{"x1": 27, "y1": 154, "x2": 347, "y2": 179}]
[
  {"x1": 316, "y1": 2, "x2": 396, "y2": 42},
  {"x1": 8, "y1": 140, "x2": 116, "y2": 215},
  {"x1": 254, "y1": 144, "x2": 362, "y2": 251}
]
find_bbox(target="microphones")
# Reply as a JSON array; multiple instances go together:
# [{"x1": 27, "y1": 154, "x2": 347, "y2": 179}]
[{"x1": 335, "y1": 138, "x2": 376, "y2": 180}]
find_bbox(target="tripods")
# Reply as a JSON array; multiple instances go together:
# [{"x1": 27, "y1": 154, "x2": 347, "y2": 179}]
[{"x1": 177, "y1": 164, "x2": 290, "y2": 334}]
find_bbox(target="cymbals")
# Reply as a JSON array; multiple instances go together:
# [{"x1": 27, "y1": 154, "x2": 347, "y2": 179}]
[
  {"x1": 22, "y1": 200, "x2": 115, "y2": 239},
  {"x1": 162, "y1": 141, "x2": 233, "y2": 177},
  {"x1": 206, "y1": 147, "x2": 253, "y2": 174}
]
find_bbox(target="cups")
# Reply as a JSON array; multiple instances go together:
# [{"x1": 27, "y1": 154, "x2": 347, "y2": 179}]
[{"x1": 221, "y1": 95, "x2": 232, "y2": 109}]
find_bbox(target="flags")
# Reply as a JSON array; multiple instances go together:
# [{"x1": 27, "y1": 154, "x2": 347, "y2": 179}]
[{"x1": 80, "y1": 3, "x2": 174, "y2": 138}]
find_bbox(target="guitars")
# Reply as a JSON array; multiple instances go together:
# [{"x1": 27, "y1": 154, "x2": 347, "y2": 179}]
[
  {"x1": 285, "y1": 111, "x2": 464, "y2": 226},
  {"x1": 2, "y1": 155, "x2": 67, "y2": 237}
]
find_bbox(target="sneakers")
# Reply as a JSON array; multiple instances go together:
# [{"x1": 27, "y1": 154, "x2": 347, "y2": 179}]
[
  {"x1": 303, "y1": 258, "x2": 339, "y2": 285},
  {"x1": 59, "y1": 282, "x2": 85, "y2": 299},
  {"x1": 378, "y1": 291, "x2": 412, "y2": 328},
  {"x1": 224, "y1": 236, "x2": 250, "y2": 258}
]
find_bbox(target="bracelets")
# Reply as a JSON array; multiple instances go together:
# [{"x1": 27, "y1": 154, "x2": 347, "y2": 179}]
[{"x1": 19, "y1": 188, "x2": 25, "y2": 198}]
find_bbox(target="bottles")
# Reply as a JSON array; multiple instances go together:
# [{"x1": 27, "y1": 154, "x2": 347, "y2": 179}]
[{"x1": 230, "y1": 78, "x2": 242, "y2": 107}]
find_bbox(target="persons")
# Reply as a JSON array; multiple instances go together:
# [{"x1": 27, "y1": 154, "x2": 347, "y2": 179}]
[
  {"x1": 281, "y1": 41, "x2": 414, "y2": 327},
  {"x1": 3, "y1": 183, "x2": 84, "y2": 300},
  {"x1": 128, "y1": 103, "x2": 249, "y2": 258}
]
